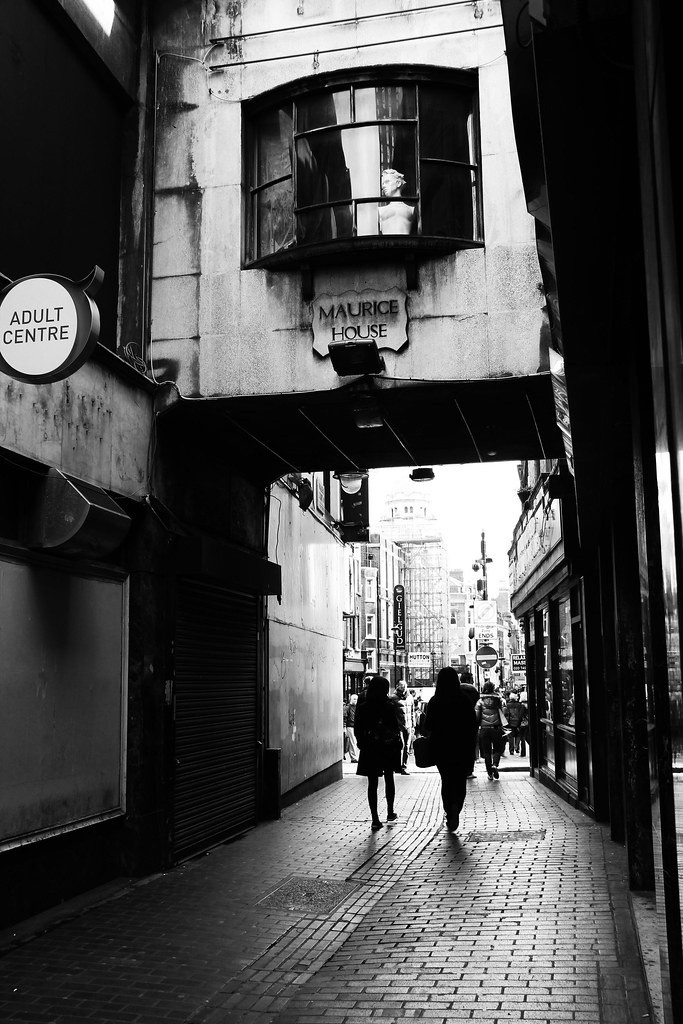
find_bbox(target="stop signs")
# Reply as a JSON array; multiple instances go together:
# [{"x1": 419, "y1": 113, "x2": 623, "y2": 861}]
[{"x1": 474, "y1": 646, "x2": 499, "y2": 670}]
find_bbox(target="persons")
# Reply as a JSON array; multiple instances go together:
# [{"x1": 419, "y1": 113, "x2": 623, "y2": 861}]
[
  {"x1": 343, "y1": 667, "x2": 529, "y2": 779},
  {"x1": 420, "y1": 668, "x2": 480, "y2": 832},
  {"x1": 353, "y1": 675, "x2": 402, "y2": 830},
  {"x1": 377, "y1": 169, "x2": 415, "y2": 235},
  {"x1": 474, "y1": 680, "x2": 503, "y2": 780}
]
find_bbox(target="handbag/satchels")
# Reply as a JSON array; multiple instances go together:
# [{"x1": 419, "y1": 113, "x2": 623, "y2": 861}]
[{"x1": 413, "y1": 737, "x2": 435, "y2": 768}]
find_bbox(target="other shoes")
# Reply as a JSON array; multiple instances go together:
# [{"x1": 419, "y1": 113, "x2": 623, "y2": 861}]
[
  {"x1": 351, "y1": 760, "x2": 358, "y2": 763},
  {"x1": 400, "y1": 770, "x2": 410, "y2": 775},
  {"x1": 510, "y1": 750, "x2": 514, "y2": 755},
  {"x1": 516, "y1": 750, "x2": 519, "y2": 753},
  {"x1": 491, "y1": 765, "x2": 499, "y2": 779},
  {"x1": 467, "y1": 775, "x2": 477, "y2": 778},
  {"x1": 489, "y1": 775, "x2": 493, "y2": 780},
  {"x1": 446, "y1": 807, "x2": 459, "y2": 831},
  {"x1": 372, "y1": 820, "x2": 382, "y2": 828},
  {"x1": 387, "y1": 813, "x2": 397, "y2": 820}
]
